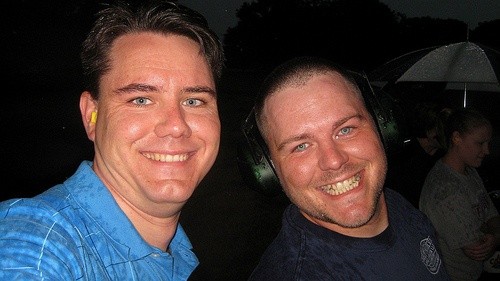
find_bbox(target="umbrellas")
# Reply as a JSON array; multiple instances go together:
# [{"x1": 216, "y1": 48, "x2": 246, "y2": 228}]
[{"x1": 380, "y1": 42, "x2": 496, "y2": 106}]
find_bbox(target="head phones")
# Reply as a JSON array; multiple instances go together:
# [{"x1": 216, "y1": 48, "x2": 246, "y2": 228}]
[{"x1": 240, "y1": 61, "x2": 402, "y2": 203}]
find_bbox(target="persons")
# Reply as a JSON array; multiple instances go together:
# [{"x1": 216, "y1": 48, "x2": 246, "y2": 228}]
[
  {"x1": 392, "y1": 102, "x2": 500, "y2": 280},
  {"x1": 1, "y1": 1, "x2": 223, "y2": 281},
  {"x1": 245, "y1": 56, "x2": 448, "y2": 281}
]
